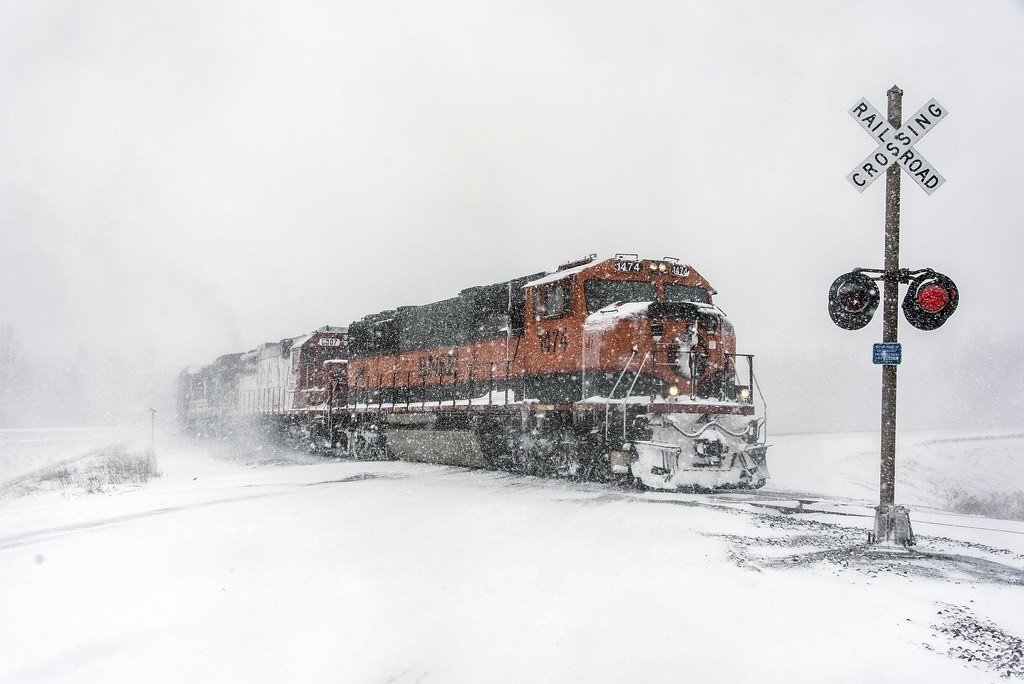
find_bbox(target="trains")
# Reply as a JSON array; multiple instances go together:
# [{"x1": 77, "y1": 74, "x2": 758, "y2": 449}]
[{"x1": 173, "y1": 253, "x2": 772, "y2": 496}]
